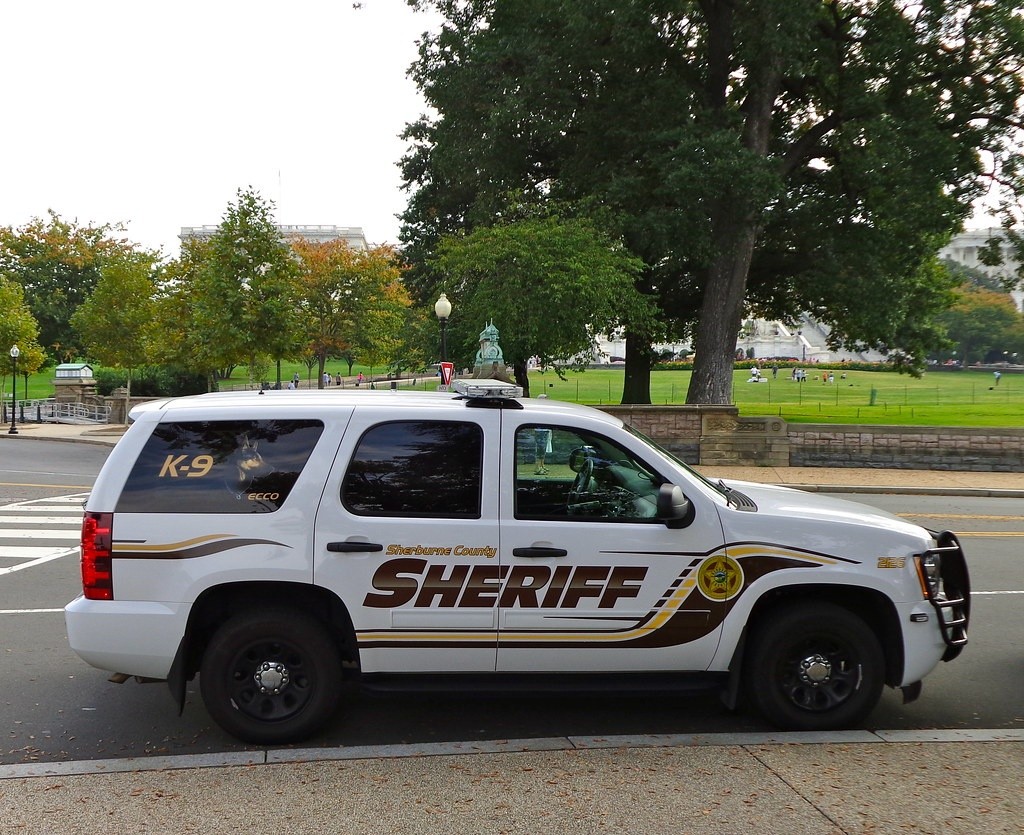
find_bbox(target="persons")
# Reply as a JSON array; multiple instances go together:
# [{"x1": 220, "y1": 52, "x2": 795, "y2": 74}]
[
  {"x1": 792, "y1": 366, "x2": 806, "y2": 383},
  {"x1": 773, "y1": 363, "x2": 779, "y2": 379},
  {"x1": 288, "y1": 372, "x2": 299, "y2": 391},
  {"x1": 823, "y1": 370, "x2": 833, "y2": 385},
  {"x1": 357, "y1": 372, "x2": 363, "y2": 384},
  {"x1": 816, "y1": 359, "x2": 819, "y2": 363},
  {"x1": 842, "y1": 358, "x2": 852, "y2": 362},
  {"x1": 323, "y1": 371, "x2": 341, "y2": 387},
  {"x1": 533, "y1": 394, "x2": 552, "y2": 475},
  {"x1": 750, "y1": 366, "x2": 762, "y2": 381},
  {"x1": 993, "y1": 370, "x2": 1001, "y2": 386},
  {"x1": 841, "y1": 371, "x2": 846, "y2": 379}
]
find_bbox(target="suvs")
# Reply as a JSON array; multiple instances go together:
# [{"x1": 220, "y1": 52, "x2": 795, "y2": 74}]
[{"x1": 62, "y1": 378, "x2": 970, "y2": 730}]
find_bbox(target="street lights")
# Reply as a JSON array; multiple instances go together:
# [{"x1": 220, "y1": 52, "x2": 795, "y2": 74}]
[
  {"x1": 435, "y1": 293, "x2": 451, "y2": 392},
  {"x1": 8, "y1": 344, "x2": 19, "y2": 434}
]
